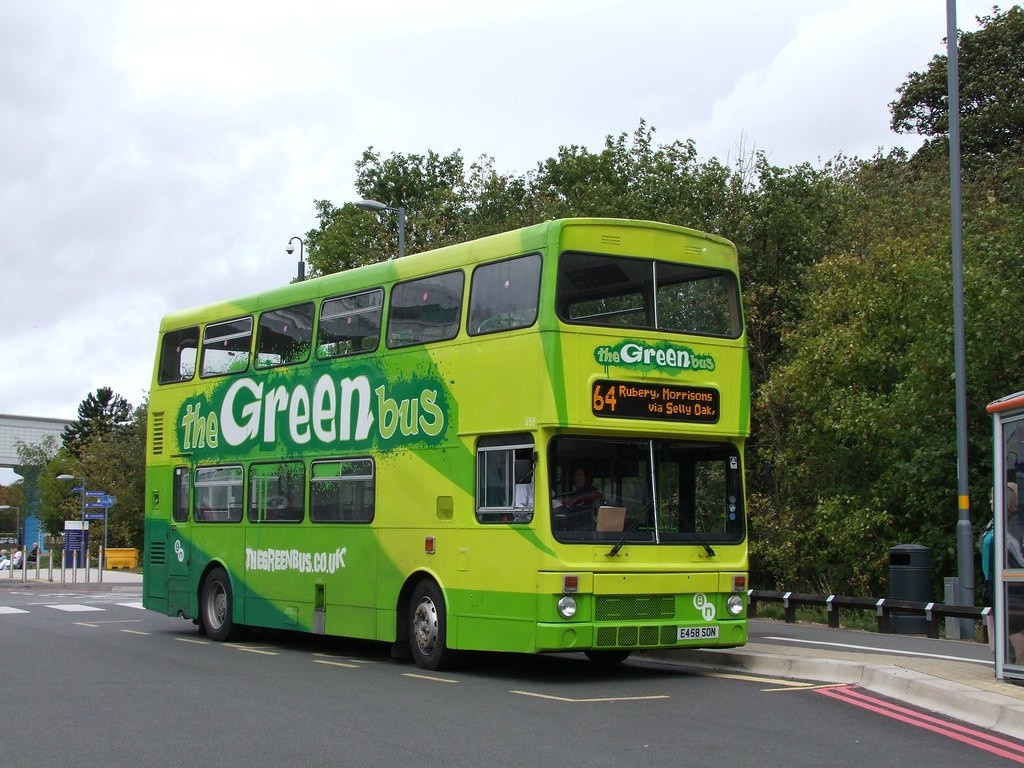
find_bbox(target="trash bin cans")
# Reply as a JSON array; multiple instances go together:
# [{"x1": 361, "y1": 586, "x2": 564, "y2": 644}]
[
  {"x1": 888, "y1": 544, "x2": 935, "y2": 634},
  {"x1": 105, "y1": 547, "x2": 140, "y2": 570}
]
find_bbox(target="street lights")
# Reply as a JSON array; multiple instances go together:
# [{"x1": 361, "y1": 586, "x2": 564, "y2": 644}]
[
  {"x1": 56, "y1": 474, "x2": 86, "y2": 568},
  {"x1": 285, "y1": 236, "x2": 305, "y2": 282},
  {"x1": 356, "y1": 200, "x2": 406, "y2": 257},
  {"x1": 0, "y1": 505, "x2": 21, "y2": 545}
]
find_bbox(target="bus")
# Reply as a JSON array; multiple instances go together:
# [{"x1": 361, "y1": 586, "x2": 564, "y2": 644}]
[{"x1": 140, "y1": 216, "x2": 780, "y2": 673}]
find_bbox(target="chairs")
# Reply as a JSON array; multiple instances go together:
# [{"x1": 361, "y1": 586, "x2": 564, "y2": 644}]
[{"x1": 6, "y1": 556, "x2": 24, "y2": 569}]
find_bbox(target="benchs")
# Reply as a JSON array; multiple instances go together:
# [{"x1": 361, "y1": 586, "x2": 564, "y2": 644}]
[
  {"x1": 27, "y1": 561, "x2": 37, "y2": 569},
  {"x1": 177, "y1": 500, "x2": 374, "y2": 521}
]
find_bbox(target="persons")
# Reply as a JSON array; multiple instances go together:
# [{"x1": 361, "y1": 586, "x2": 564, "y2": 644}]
[
  {"x1": 979, "y1": 482, "x2": 1024, "y2": 666},
  {"x1": 0, "y1": 543, "x2": 39, "y2": 570},
  {"x1": 513, "y1": 464, "x2": 609, "y2": 523}
]
